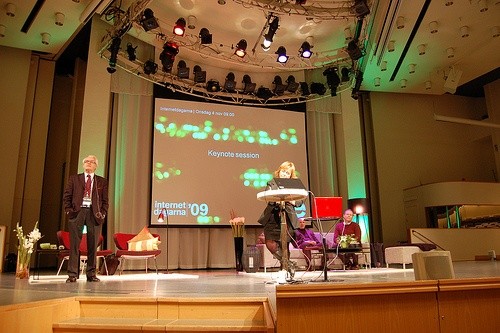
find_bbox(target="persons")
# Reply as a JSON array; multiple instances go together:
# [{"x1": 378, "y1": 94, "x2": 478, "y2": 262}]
[
  {"x1": 65, "y1": 155, "x2": 108, "y2": 283},
  {"x1": 257, "y1": 161, "x2": 362, "y2": 277}
]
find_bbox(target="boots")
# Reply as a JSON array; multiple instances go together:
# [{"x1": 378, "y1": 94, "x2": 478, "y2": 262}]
[{"x1": 273, "y1": 251, "x2": 297, "y2": 282}]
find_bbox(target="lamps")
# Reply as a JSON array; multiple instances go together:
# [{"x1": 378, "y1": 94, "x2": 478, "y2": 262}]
[
  {"x1": 158, "y1": 208, "x2": 171, "y2": 274},
  {"x1": 0, "y1": 0, "x2": 500, "y2": 100}
]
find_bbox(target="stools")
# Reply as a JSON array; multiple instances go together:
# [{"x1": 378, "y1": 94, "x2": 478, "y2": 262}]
[{"x1": 385, "y1": 246, "x2": 420, "y2": 269}]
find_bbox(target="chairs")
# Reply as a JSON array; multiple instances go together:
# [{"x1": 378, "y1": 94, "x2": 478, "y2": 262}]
[
  {"x1": 113, "y1": 231, "x2": 161, "y2": 276},
  {"x1": 56, "y1": 230, "x2": 113, "y2": 278}
]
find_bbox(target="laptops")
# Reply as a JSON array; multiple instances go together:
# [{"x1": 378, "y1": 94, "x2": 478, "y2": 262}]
[
  {"x1": 274, "y1": 178, "x2": 306, "y2": 190},
  {"x1": 311, "y1": 197, "x2": 343, "y2": 219}
]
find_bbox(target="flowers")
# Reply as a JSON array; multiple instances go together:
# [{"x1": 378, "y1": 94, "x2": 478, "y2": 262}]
[{"x1": 12, "y1": 220, "x2": 44, "y2": 279}]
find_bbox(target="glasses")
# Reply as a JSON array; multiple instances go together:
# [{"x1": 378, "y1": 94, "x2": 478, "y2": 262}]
[{"x1": 84, "y1": 160, "x2": 97, "y2": 164}]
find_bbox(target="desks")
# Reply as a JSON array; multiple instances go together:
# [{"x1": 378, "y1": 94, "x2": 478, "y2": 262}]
[
  {"x1": 257, "y1": 189, "x2": 309, "y2": 286},
  {"x1": 33, "y1": 248, "x2": 69, "y2": 280},
  {"x1": 305, "y1": 218, "x2": 345, "y2": 282}
]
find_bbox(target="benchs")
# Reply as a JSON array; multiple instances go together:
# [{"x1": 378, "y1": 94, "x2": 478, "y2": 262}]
[{"x1": 257, "y1": 231, "x2": 372, "y2": 272}]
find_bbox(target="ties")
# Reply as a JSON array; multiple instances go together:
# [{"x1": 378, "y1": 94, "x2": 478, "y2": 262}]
[{"x1": 85, "y1": 175, "x2": 92, "y2": 198}]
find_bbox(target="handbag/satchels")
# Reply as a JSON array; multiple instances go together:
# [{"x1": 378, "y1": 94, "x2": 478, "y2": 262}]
[
  {"x1": 101, "y1": 254, "x2": 121, "y2": 274},
  {"x1": 3, "y1": 253, "x2": 17, "y2": 272}
]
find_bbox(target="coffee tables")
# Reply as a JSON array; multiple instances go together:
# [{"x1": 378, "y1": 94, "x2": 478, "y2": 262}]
[{"x1": 320, "y1": 247, "x2": 363, "y2": 271}]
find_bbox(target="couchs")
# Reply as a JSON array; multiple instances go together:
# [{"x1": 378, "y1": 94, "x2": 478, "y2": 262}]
[{"x1": 126, "y1": 226, "x2": 161, "y2": 251}]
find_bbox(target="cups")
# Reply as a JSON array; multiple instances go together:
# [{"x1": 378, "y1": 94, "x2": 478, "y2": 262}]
[{"x1": 59, "y1": 246, "x2": 64, "y2": 250}]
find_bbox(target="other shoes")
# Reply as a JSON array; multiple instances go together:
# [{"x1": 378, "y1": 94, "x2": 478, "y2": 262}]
[
  {"x1": 87, "y1": 275, "x2": 100, "y2": 281},
  {"x1": 306, "y1": 266, "x2": 311, "y2": 271},
  {"x1": 320, "y1": 266, "x2": 330, "y2": 271},
  {"x1": 66, "y1": 276, "x2": 76, "y2": 283},
  {"x1": 345, "y1": 262, "x2": 359, "y2": 270}
]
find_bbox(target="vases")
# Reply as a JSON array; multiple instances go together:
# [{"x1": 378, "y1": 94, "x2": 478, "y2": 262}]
[{"x1": 16, "y1": 249, "x2": 30, "y2": 279}]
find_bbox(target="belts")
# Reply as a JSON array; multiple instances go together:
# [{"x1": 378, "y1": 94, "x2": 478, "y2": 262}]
[{"x1": 79, "y1": 205, "x2": 92, "y2": 208}]
